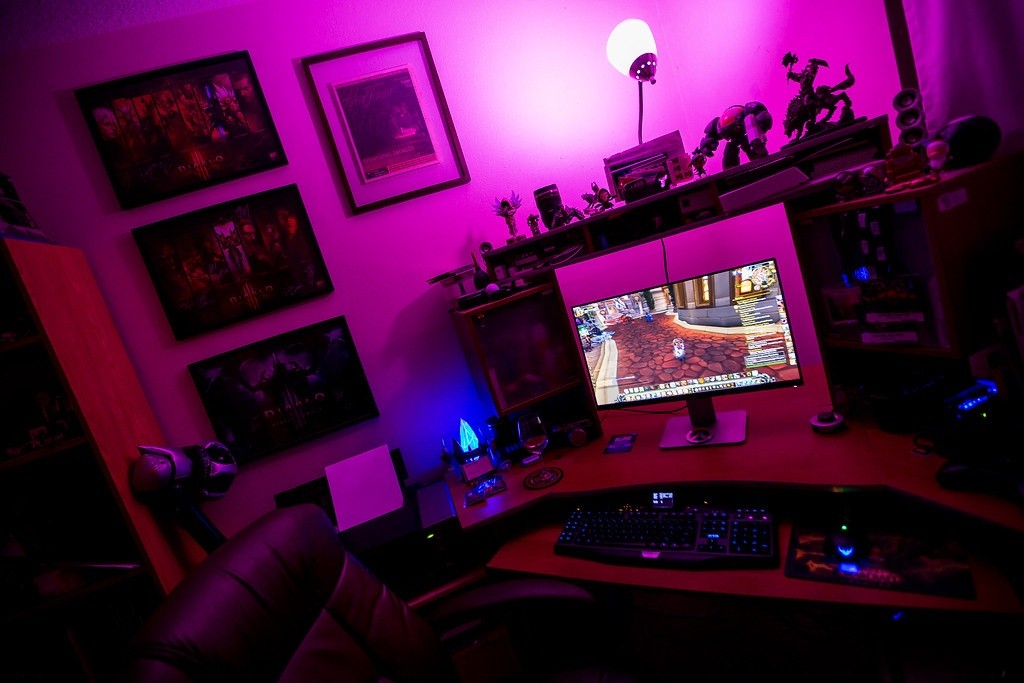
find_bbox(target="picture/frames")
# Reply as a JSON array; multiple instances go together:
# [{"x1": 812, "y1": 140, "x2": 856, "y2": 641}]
[
  {"x1": 188, "y1": 315, "x2": 379, "y2": 466},
  {"x1": 76, "y1": 50, "x2": 291, "y2": 210},
  {"x1": 130, "y1": 182, "x2": 334, "y2": 341},
  {"x1": 302, "y1": 30, "x2": 471, "y2": 214}
]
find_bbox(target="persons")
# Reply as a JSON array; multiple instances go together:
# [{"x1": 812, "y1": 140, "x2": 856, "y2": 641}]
[
  {"x1": 499, "y1": 200, "x2": 517, "y2": 235},
  {"x1": 687, "y1": 147, "x2": 708, "y2": 179},
  {"x1": 594, "y1": 188, "x2": 618, "y2": 211}
]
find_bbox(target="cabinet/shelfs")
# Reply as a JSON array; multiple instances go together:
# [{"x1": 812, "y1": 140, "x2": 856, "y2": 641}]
[
  {"x1": 450, "y1": 149, "x2": 1024, "y2": 683},
  {"x1": 0, "y1": 239, "x2": 209, "y2": 683}
]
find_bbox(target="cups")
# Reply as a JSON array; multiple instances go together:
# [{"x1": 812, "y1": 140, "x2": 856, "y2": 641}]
[{"x1": 533, "y1": 184, "x2": 567, "y2": 230}]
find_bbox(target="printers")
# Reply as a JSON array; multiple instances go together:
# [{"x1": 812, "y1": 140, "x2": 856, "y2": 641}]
[{"x1": 272, "y1": 450, "x2": 431, "y2": 603}]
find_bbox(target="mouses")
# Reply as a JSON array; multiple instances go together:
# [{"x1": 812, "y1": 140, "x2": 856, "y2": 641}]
[{"x1": 824, "y1": 523, "x2": 859, "y2": 563}]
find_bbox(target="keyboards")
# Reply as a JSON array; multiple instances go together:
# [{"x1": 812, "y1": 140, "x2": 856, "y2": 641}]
[{"x1": 553, "y1": 507, "x2": 781, "y2": 571}]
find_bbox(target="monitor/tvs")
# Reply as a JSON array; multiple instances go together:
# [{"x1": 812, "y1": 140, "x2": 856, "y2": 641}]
[{"x1": 570, "y1": 257, "x2": 805, "y2": 448}]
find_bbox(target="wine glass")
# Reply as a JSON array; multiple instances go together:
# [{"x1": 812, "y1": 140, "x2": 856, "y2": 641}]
[{"x1": 518, "y1": 412, "x2": 557, "y2": 486}]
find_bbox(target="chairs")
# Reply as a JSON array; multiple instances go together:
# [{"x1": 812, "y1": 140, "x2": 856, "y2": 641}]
[{"x1": 133, "y1": 501, "x2": 596, "y2": 683}]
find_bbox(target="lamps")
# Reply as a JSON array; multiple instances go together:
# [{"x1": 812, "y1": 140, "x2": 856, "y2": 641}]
[{"x1": 606, "y1": 17, "x2": 659, "y2": 146}]
